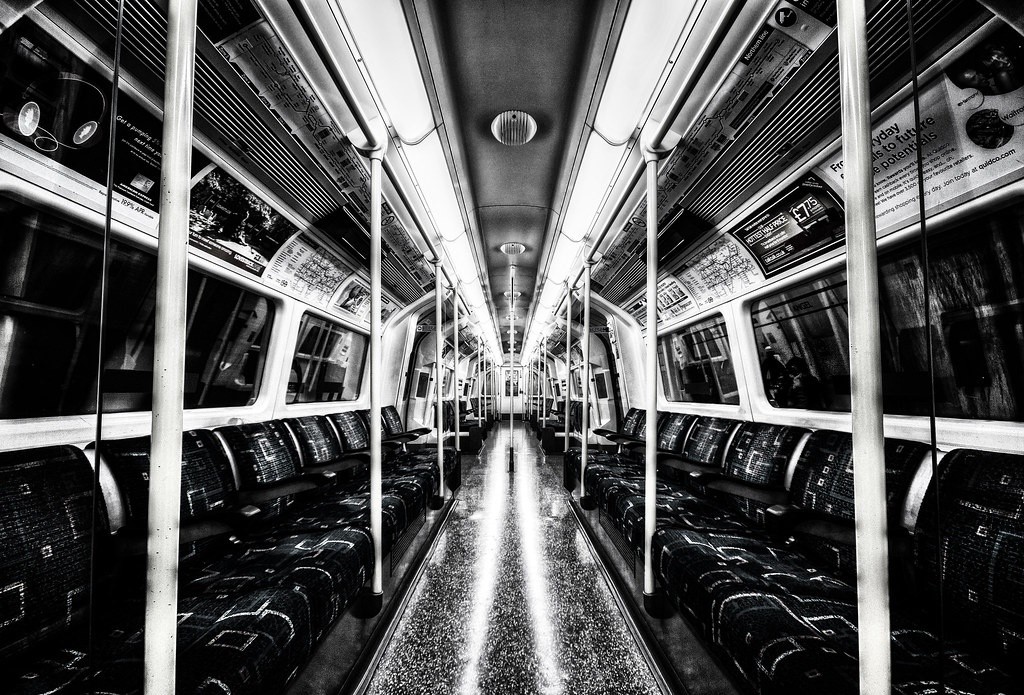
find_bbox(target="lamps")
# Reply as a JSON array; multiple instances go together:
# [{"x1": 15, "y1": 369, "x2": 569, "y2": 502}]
[
  {"x1": 500, "y1": 242, "x2": 526, "y2": 255},
  {"x1": 490, "y1": 110, "x2": 538, "y2": 147}
]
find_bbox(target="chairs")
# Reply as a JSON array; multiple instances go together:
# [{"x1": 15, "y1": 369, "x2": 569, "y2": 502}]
[{"x1": 0, "y1": 397, "x2": 1023, "y2": 695}]
